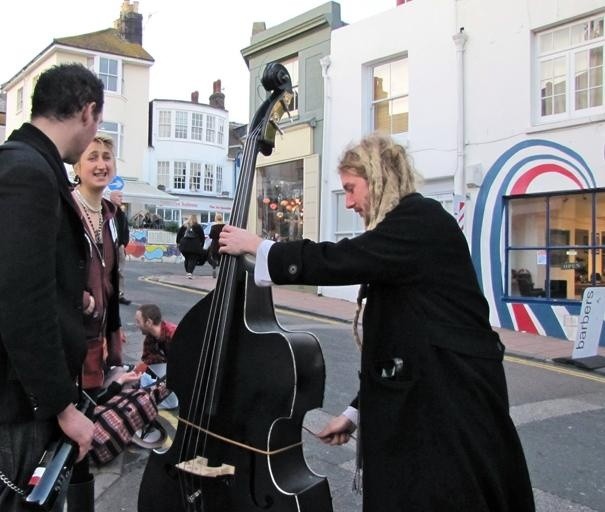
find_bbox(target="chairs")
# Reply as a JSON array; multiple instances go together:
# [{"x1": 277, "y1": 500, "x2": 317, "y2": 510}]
[
  {"x1": 516, "y1": 278, "x2": 545, "y2": 297},
  {"x1": 518, "y1": 273, "x2": 543, "y2": 291}
]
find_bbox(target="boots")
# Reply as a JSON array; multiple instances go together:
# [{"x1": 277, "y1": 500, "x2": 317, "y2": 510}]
[{"x1": 67, "y1": 472, "x2": 94, "y2": 512}]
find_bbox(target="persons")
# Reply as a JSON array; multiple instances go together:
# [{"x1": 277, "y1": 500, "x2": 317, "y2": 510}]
[
  {"x1": 109, "y1": 189, "x2": 132, "y2": 304},
  {"x1": 208, "y1": 213, "x2": 225, "y2": 278},
  {"x1": 88, "y1": 370, "x2": 141, "y2": 420},
  {"x1": 176, "y1": 214, "x2": 205, "y2": 278},
  {"x1": 136, "y1": 305, "x2": 178, "y2": 410},
  {"x1": 0, "y1": 62, "x2": 104, "y2": 512},
  {"x1": 218, "y1": 136, "x2": 536, "y2": 512},
  {"x1": 68, "y1": 134, "x2": 121, "y2": 512}
]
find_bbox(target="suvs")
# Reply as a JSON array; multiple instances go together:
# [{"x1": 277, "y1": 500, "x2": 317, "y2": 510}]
[{"x1": 199, "y1": 222, "x2": 230, "y2": 265}]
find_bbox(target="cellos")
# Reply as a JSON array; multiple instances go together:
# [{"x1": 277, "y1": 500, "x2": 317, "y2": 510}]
[{"x1": 138, "y1": 62, "x2": 333, "y2": 511}]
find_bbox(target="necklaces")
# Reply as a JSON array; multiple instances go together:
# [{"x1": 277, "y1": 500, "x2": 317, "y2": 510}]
[
  {"x1": 77, "y1": 187, "x2": 104, "y2": 212},
  {"x1": 73, "y1": 191, "x2": 103, "y2": 246},
  {"x1": 84, "y1": 296, "x2": 96, "y2": 315}
]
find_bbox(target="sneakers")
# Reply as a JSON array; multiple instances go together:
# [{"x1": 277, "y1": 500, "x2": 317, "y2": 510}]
[
  {"x1": 187, "y1": 273, "x2": 193, "y2": 279},
  {"x1": 119, "y1": 297, "x2": 132, "y2": 306}
]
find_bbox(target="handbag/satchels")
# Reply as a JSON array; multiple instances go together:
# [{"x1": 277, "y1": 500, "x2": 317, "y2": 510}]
[{"x1": 184, "y1": 228, "x2": 198, "y2": 239}]
